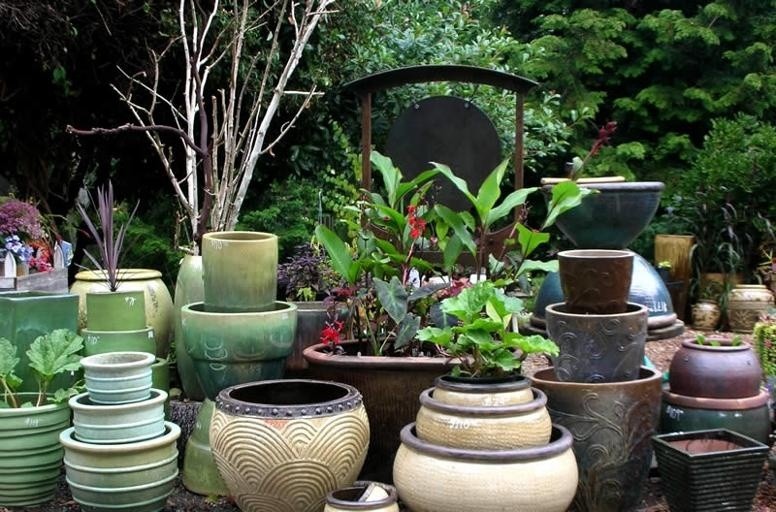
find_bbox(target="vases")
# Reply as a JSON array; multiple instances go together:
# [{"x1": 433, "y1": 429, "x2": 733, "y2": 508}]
[
  {"x1": 0, "y1": 289, "x2": 78, "y2": 393},
  {"x1": 174, "y1": 255, "x2": 212, "y2": 400},
  {"x1": 697, "y1": 273, "x2": 743, "y2": 298},
  {"x1": 181, "y1": 301, "x2": 297, "y2": 401},
  {"x1": 150, "y1": 357, "x2": 169, "y2": 420},
  {"x1": 208, "y1": 379, "x2": 370, "y2": 512},
  {"x1": 80, "y1": 326, "x2": 157, "y2": 358},
  {"x1": 301, "y1": 336, "x2": 528, "y2": 485},
  {"x1": 557, "y1": 248, "x2": 637, "y2": 313},
  {"x1": 540, "y1": 182, "x2": 665, "y2": 250},
  {"x1": 689, "y1": 299, "x2": 721, "y2": 333},
  {"x1": 201, "y1": 231, "x2": 277, "y2": 313},
  {"x1": 392, "y1": 421, "x2": 578, "y2": 512},
  {"x1": 544, "y1": 301, "x2": 648, "y2": 383},
  {"x1": 322, "y1": 481, "x2": 399, "y2": 512},
  {"x1": 286, "y1": 301, "x2": 347, "y2": 371},
  {"x1": 651, "y1": 428, "x2": 770, "y2": 512},
  {"x1": 58, "y1": 421, "x2": 181, "y2": 512},
  {"x1": 661, "y1": 385, "x2": 771, "y2": 447},
  {"x1": 181, "y1": 395, "x2": 231, "y2": 499},
  {"x1": 68, "y1": 388, "x2": 168, "y2": 445},
  {"x1": 79, "y1": 350, "x2": 156, "y2": 407},
  {"x1": 725, "y1": 284, "x2": 775, "y2": 334},
  {"x1": 527, "y1": 365, "x2": 661, "y2": 512},
  {"x1": 414, "y1": 387, "x2": 552, "y2": 451}
]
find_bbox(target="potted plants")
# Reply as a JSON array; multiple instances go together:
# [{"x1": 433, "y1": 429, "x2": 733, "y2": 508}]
[
  {"x1": 668, "y1": 333, "x2": 762, "y2": 398},
  {"x1": 415, "y1": 280, "x2": 560, "y2": 407},
  {"x1": 0, "y1": 327, "x2": 83, "y2": 509},
  {"x1": 72, "y1": 178, "x2": 155, "y2": 332}
]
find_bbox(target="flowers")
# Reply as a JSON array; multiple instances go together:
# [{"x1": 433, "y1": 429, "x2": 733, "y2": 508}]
[
  {"x1": 310, "y1": 120, "x2": 618, "y2": 356},
  {"x1": 0, "y1": 201, "x2": 54, "y2": 272}
]
